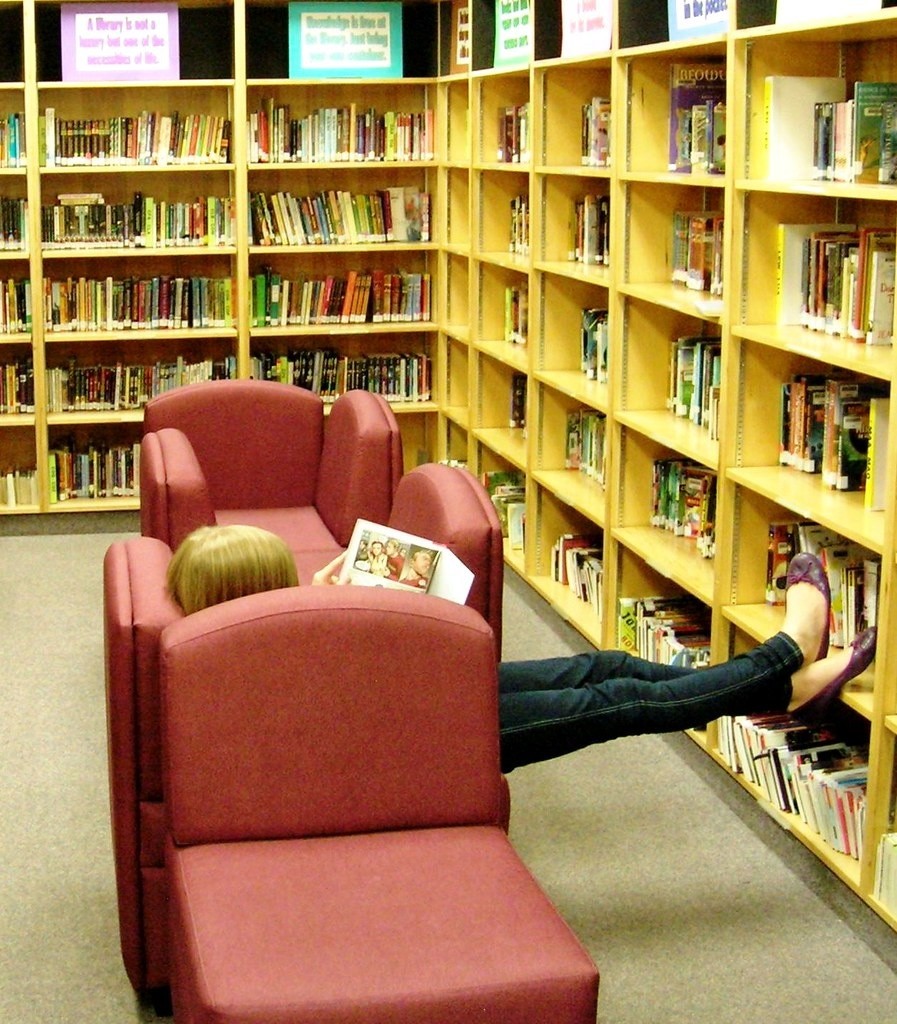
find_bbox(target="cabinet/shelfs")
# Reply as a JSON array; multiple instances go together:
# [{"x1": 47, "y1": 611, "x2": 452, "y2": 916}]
[{"x1": 1, "y1": 1, "x2": 896, "y2": 929}]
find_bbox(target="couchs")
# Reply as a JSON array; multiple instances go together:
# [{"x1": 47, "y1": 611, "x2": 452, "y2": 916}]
[{"x1": 100, "y1": 380, "x2": 602, "y2": 1023}]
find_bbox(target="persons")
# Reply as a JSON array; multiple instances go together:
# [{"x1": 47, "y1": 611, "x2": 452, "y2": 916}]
[{"x1": 164, "y1": 523, "x2": 879, "y2": 774}]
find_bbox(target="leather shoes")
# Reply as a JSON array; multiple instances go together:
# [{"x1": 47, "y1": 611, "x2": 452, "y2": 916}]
[
  {"x1": 788, "y1": 626, "x2": 877, "y2": 730},
  {"x1": 785, "y1": 553, "x2": 831, "y2": 662}
]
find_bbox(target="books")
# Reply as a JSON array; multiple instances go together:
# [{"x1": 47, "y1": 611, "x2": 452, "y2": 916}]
[{"x1": 1, "y1": 61, "x2": 896, "y2": 913}]
